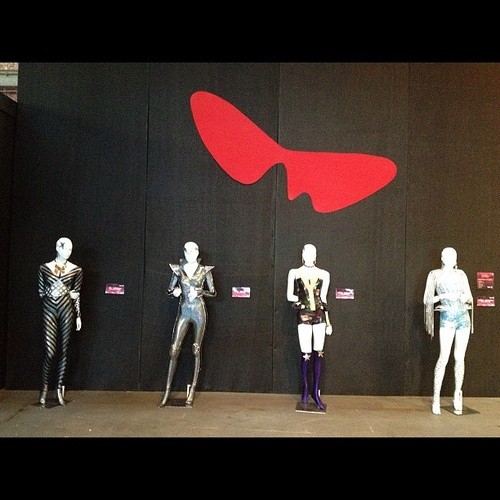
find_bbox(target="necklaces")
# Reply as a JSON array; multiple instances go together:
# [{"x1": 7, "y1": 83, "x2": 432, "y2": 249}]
[
  {"x1": 52, "y1": 258, "x2": 67, "y2": 275},
  {"x1": 303, "y1": 264, "x2": 315, "y2": 268}
]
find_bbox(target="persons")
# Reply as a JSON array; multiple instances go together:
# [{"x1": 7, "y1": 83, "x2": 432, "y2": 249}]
[
  {"x1": 287, "y1": 243, "x2": 333, "y2": 410},
  {"x1": 39, "y1": 238, "x2": 83, "y2": 405},
  {"x1": 155, "y1": 243, "x2": 217, "y2": 408},
  {"x1": 423, "y1": 248, "x2": 473, "y2": 414}
]
